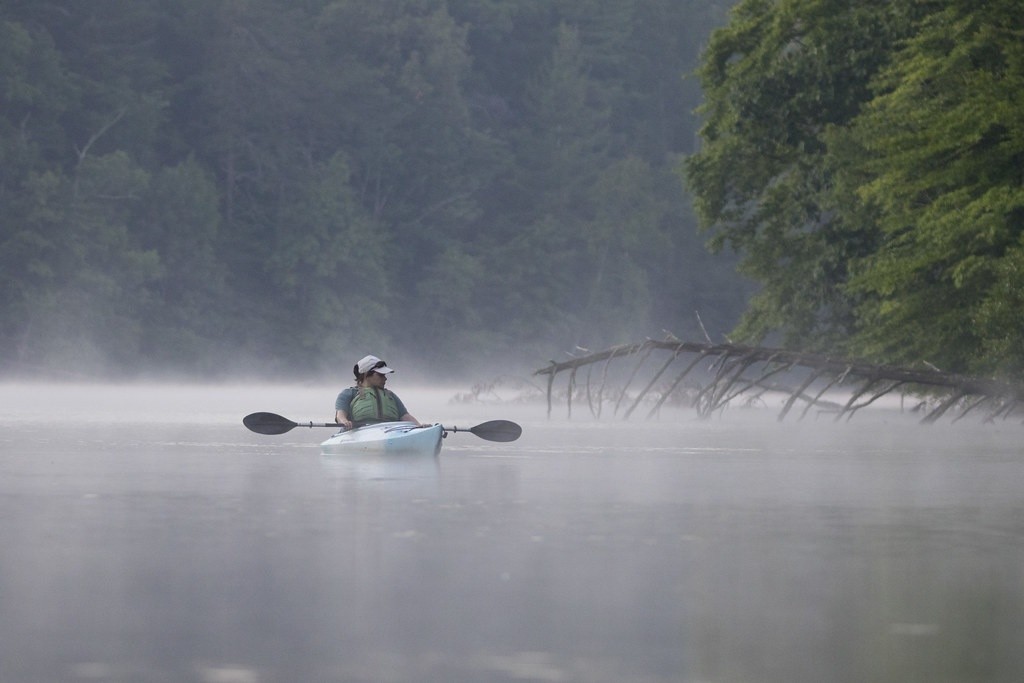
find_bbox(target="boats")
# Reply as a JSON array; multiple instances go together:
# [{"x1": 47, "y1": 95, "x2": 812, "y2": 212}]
[{"x1": 320, "y1": 421, "x2": 448, "y2": 458}]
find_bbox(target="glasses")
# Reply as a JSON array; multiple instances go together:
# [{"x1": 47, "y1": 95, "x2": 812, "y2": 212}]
[{"x1": 367, "y1": 360, "x2": 387, "y2": 372}]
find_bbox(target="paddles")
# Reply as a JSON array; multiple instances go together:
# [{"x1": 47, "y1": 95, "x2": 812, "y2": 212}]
[{"x1": 243, "y1": 411, "x2": 522, "y2": 443}]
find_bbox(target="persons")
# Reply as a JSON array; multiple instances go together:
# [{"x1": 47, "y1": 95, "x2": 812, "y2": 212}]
[{"x1": 335, "y1": 355, "x2": 433, "y2": 434}]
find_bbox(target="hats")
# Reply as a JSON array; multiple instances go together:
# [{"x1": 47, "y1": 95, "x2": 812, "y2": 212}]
[{"x1": 357, "y1": 353, "x2": 395, "y2": 376}]
